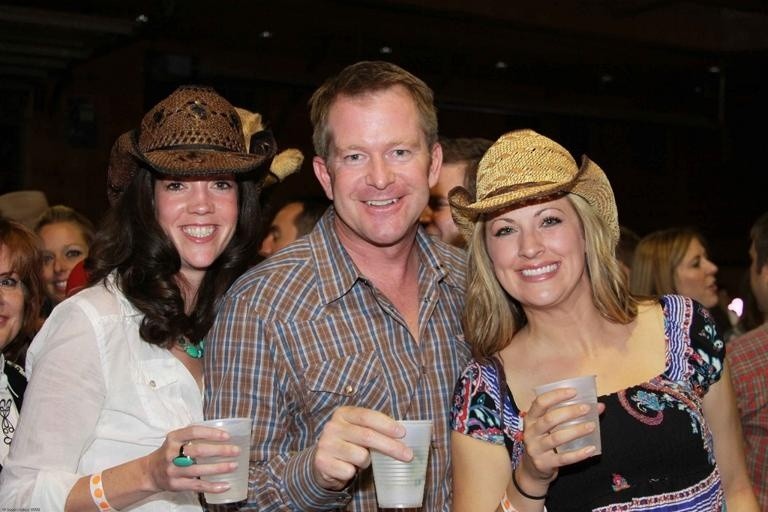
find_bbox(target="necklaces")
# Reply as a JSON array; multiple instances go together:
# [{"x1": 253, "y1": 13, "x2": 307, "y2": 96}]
[{"x1": 179, "y1": 338, "x2": 206, "y2": 359}]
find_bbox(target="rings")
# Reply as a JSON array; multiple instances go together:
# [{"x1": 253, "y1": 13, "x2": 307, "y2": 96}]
[{"x1": 174, "y1": 443, "x2": 195, "y2": 468}]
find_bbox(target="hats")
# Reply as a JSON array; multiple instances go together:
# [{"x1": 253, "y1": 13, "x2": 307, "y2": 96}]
[
  {"x1": 106, "y1": 85, "x2": 278, "y2": 208},
  {"x1": 0, "y1": 191, "x2": 49, "y2": 227},
  {"x1": 449, "y1": 129, "x2": 622, "y2": 245}
]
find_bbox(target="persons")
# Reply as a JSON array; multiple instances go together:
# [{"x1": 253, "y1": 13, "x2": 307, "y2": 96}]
[
  {"x1": 261, "y1": 200, "x2": 327, "y2": 262},
  {"x1": 451, "y1": 129, "x2": 759, "y2": 511},
  {"x1": 1, "y1": 82, "x2": 266, "y2": 512},
  {"x1": 726, "y1": 213, "x2": 768, "y2": 511},
  {"x1": 635, "y1": 231, "x2": 722, "y2": 307},
  {"x1": 204, "y1": 59, "x2": 472, "y2": 512},
  {"x1": 421, "y1": 139, "x2": 491, "y2": 251},
  {"x1": 0, "y1": 216, "x2": 46, "y2": 476},
  {"x1": 34, "y1": 205, "x2": 95, "y2": 302}
]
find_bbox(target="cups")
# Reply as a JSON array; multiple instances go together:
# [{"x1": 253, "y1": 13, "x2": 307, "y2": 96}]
[
  {"x1": 369, "y1": 419, "x2": 434, "y2": 511},
  {"x1": 191, "y1": 419, "x2": 252, "y2": 504},
  {"x1": 531, "y1": 373, "x2": 602, "y2": 457}
]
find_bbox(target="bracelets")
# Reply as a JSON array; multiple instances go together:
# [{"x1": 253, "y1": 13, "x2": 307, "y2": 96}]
[
  {"x1": 88, "y1": 470, "x2": 119, "y2": 511},
  {"x1": 498, "y1": 491, "x2": 548, "y2": 511}
]
